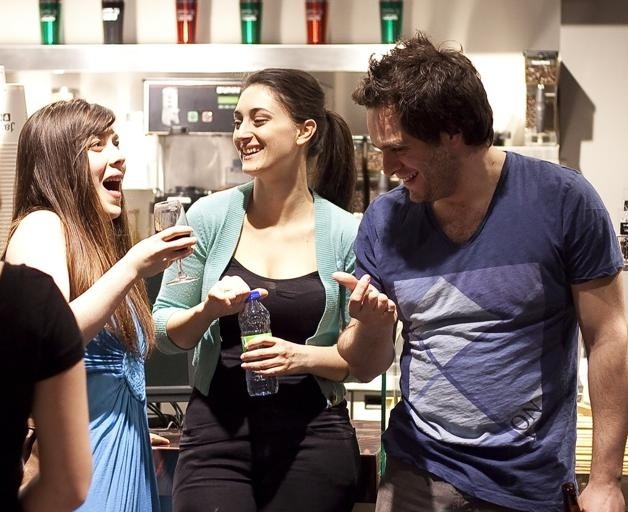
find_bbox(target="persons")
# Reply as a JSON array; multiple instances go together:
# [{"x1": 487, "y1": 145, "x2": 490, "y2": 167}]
[
  {"x1": 150, "y1": 67, "x2": 364, "y2": 512},
  {"x1": 0, "y1": 99, "x2": 199, "y2": 512},
  {"x1": 331, "y1": 29, "x2": 628, "y2": 512},
  {"x1": 0, "y1": 262, "x2": 93, "y2": 512}
]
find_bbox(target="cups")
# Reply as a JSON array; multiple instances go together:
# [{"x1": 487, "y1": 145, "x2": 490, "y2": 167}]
[
  {"x1": 38, "y1": 0, "x2": 61, "y2": 45},
  {"x1": 306, "y1": 0, "x2": 327, "y2": 44},
  {"x1": 101, "y1": 0, "x2": 124, "y2": 43},
  {"x1": 240, "y1": 0, "x2": 262, "y2": 43},
  {"x1": 0, "y1": 81, "x2": 28, "y2": 261},
  {"x1": 379, "y1": 0, "x2": 403, "y2": 44},
  {"x1": 175, "y1": 0, "x2": 197, "y2": 43}
]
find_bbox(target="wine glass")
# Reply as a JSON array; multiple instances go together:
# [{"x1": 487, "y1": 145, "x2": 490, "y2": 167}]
[{"x1": 153, "y1": 201, "x2": 200, "y2": 286}]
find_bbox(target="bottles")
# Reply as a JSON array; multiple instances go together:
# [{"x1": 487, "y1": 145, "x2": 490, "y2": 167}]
[{"x1": 238, "y1": 291, "x2": 279, "y2": 398}]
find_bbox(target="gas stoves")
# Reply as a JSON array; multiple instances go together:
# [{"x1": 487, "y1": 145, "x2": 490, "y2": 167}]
[{"x1": 150, "y1": 185, "x2": 218, "y2": 212}]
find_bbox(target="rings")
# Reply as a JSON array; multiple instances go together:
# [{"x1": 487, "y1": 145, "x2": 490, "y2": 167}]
[
  {"x1": 348, "y1": 312, "x2": 360, "y2": 317},
  {"x1": 387, "y1": 310, "x2": 394, "y2": 313}
]
